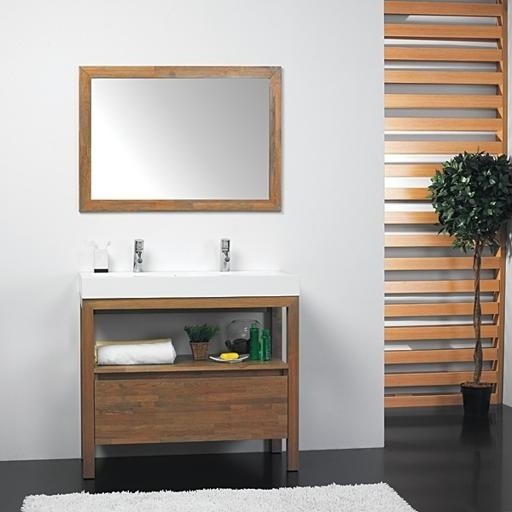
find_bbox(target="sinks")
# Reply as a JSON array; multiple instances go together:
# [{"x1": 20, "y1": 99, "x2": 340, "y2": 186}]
[
  {"x1": 174, "y1": 272, "x2": 300, "y2": 299},
  {"x1": 79, "y1": 271, "x2": 177, "y2": 299}
]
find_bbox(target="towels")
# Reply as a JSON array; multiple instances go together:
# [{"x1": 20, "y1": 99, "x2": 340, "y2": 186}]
[
  {"x1": 95, "y1": 338, "x2": 172, "y2": 362},
  {"x1": 97, "y1": 342, "x2": 177, "y2": 365}
]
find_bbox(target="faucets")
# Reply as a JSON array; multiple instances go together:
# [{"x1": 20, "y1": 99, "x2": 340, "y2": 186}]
[
  {"x1": 219, "y1": 240, "x2": 231, "y2": 272},
  {"x1": 133, "y1": 239, "x2": 144, "y2": 272}
]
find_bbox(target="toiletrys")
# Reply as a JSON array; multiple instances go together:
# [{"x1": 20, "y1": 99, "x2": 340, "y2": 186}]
[{"x1": 250, "y1": 322, "x2": 259, "y2": 360}]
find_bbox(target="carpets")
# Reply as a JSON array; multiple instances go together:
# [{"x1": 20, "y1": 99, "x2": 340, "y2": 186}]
[{"x1": 20, "y1": 481, "x2": 418, "y2": 512}]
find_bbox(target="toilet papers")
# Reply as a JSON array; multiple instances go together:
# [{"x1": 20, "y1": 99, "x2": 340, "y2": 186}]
[{"x1": 94, "y1": 240, "x2": 110, "y2": 272}]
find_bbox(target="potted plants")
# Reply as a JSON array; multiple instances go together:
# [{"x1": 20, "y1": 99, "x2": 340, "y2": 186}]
[
  {"x1": 183, "y1": 323, "x2": 221, "y2": 361},
  {"x1": 426, "y1": 146, "x2": 512, "y2": 419}
]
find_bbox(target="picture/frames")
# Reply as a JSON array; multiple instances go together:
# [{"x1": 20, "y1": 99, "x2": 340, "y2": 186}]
[{"x1": 78, "y1": 66, "x2": 282, "y2": 211}]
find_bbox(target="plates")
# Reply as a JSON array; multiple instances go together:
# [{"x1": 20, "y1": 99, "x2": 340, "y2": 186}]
[{"x1": 209, "y1": 352, "x2": 250, "y2": 363}]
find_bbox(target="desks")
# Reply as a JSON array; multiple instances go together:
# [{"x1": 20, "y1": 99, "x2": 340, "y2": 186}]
[{"x1": 81, "y1": 297, "x2": 299, "y2": 479}]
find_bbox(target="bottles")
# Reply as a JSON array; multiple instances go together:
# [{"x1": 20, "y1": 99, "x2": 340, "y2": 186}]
[{"x1": 249, "y1": 322, "x2": 260, "y2": 360}]
